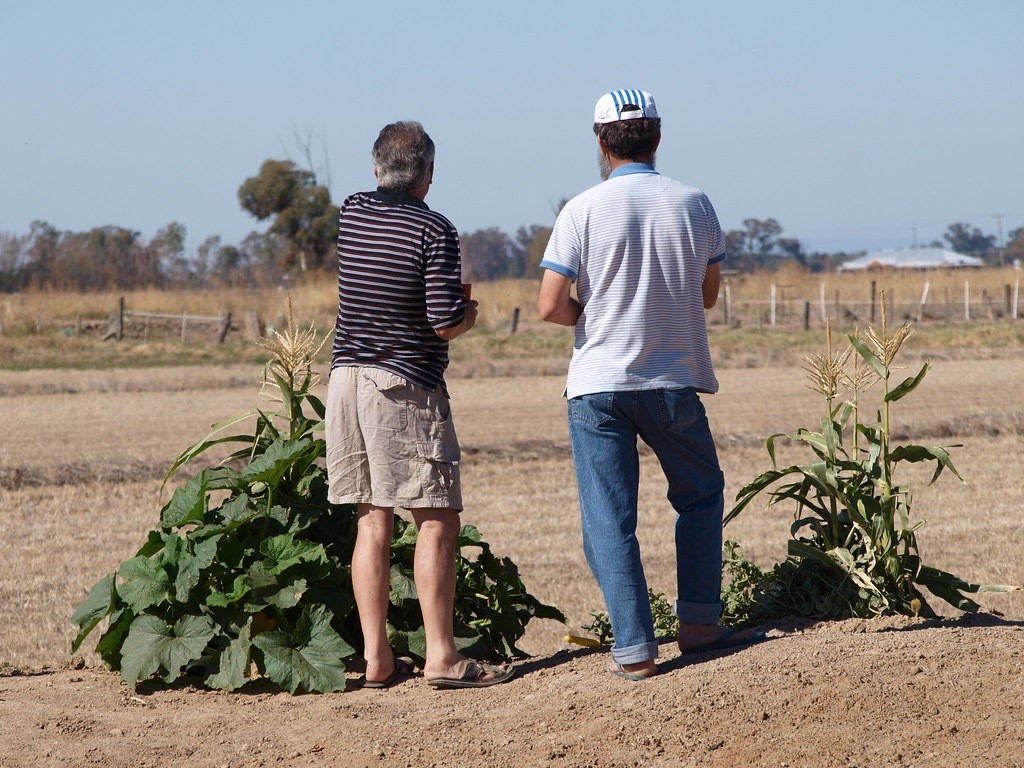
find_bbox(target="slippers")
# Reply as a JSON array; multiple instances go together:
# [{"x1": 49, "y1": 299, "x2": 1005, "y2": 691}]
[
  {"x1": 682, "y1": 628, "x2": 766, "y2": 655},
  {"x1": 427, "y1": 662, "x2": 516, "y2": 688},
  {"x1": 608, "y1": 659, "x2": 645, "y2": 680},
  {"x1": 359, "y1": 656, "x2": 414, "y2": 688}
]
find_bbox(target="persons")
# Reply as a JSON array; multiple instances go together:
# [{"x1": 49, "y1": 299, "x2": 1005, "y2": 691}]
[
  {"x1": 535, "y1": 87, "x2": 770, "y2": 679},
  {"x1": 325, "y1": 120, "x2": 518, "y2": 690}
]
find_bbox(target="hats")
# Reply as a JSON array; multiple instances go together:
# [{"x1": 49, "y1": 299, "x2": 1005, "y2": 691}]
[{"x1": 593, "y1": 89, "x2": 658, "y2": 124}]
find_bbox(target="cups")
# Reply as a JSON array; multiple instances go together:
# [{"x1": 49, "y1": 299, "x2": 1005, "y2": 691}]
[{"x1": 461, "y1": 284, "x2": 472, "y2": 301}]
